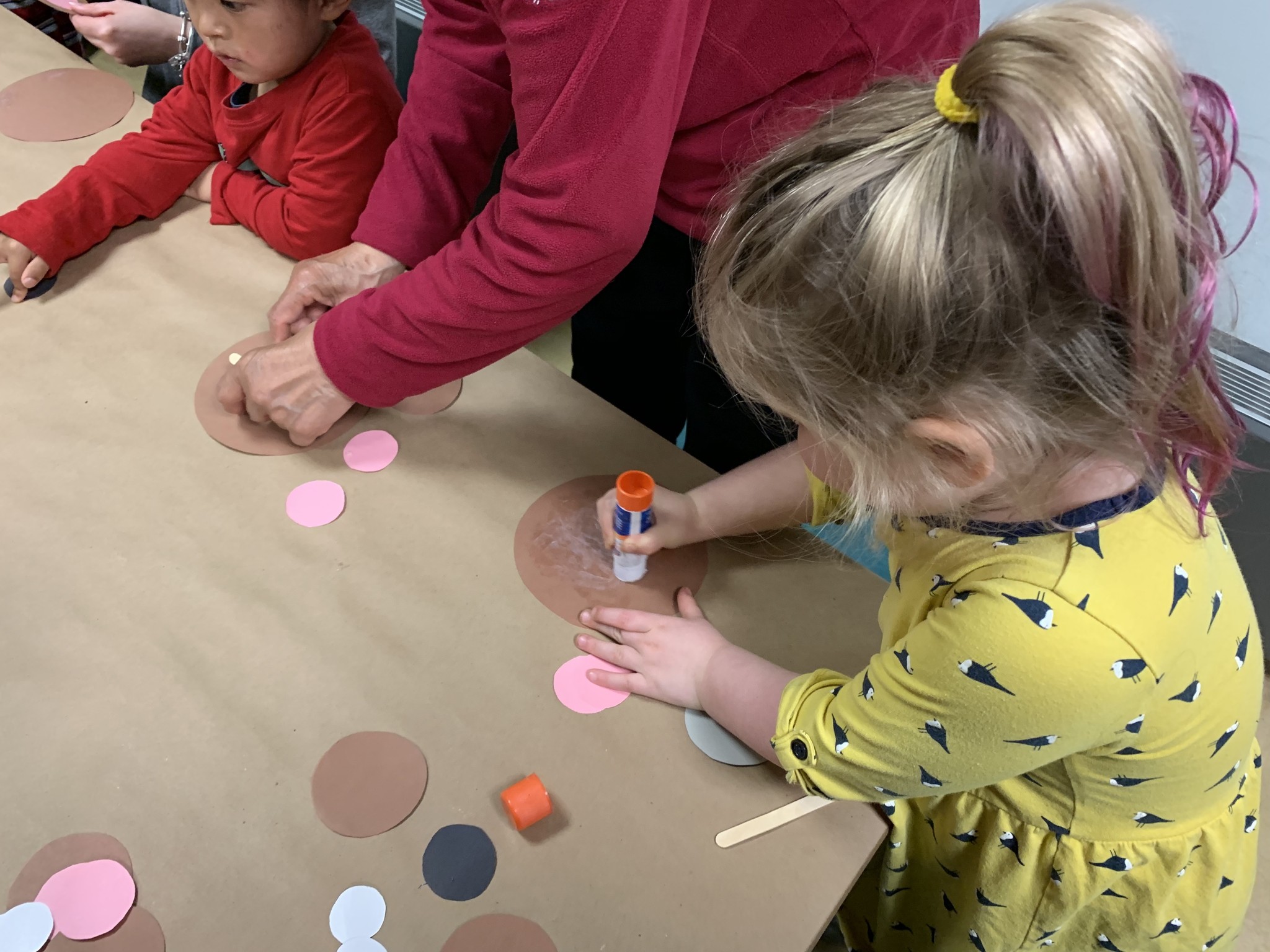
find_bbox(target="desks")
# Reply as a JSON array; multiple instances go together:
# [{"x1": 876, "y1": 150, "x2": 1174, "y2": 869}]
[{"x1": 0, "y1": 5, "x2": 890, "y2": 951}]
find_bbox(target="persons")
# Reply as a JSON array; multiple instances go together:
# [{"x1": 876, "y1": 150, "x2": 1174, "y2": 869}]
[
  {"x1": 0, "y1": 0, "x2": 407, "y2": 304},
  {"x1": 219, "y1": 0, "x2": 985, "y2": 476},
  {"x1": 70, "y1": 0, "x2": 394, "y2": 105},
  {"x1": 597, "y1": 0, "x2": 1270, "y2": 951}
]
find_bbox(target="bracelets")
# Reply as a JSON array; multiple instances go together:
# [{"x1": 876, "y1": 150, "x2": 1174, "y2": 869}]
[{"x1": 168, "y1": 10, "x2": 195, "y2": 78}]
